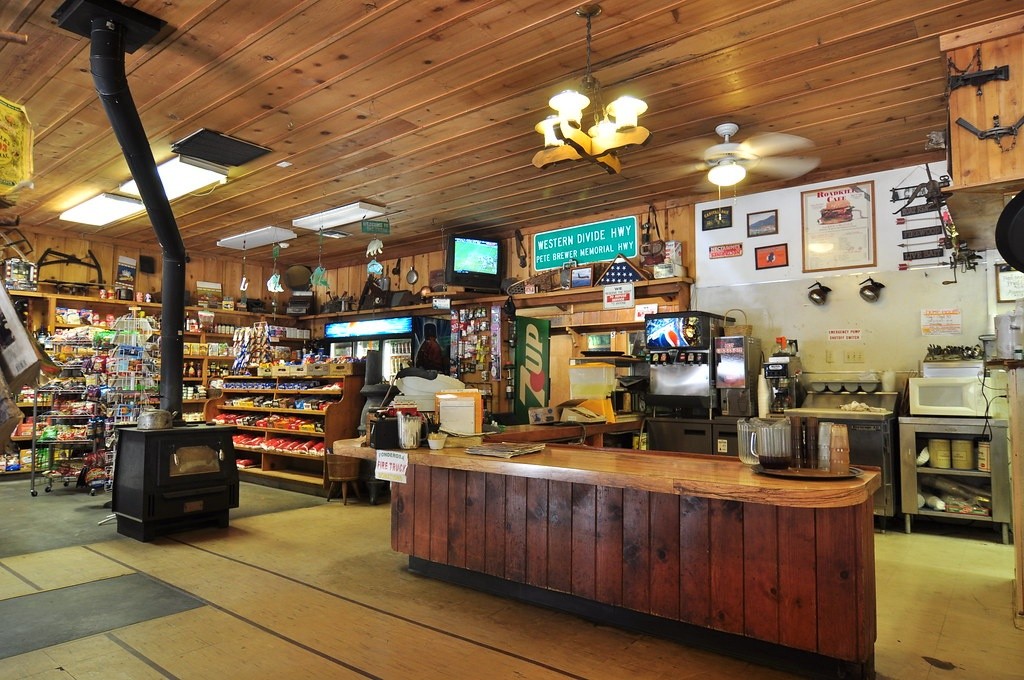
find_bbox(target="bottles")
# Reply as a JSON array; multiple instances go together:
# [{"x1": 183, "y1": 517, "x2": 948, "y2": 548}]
[
  {"x1": 183, "y1": 361, "x2": 229, "y2": 377},
  {"x1": 416, "y1": 322, "x2": 444, "y2": 376}
]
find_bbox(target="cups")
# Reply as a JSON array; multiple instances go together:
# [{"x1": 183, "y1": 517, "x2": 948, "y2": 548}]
[
  {"x1": 736, "y1": 418, "x2": 768, "y2": 465},
  {"x1": 750, "y1": 427, "x2": 792, "y2": 469},
  {"x1": 817, "y1": 421, "x2": 850, "y2": 475}
]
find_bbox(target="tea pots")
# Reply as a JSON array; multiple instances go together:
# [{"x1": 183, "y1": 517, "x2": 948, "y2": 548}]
[
  {"x1": 137, "y1": 395, "x2": 178, "y2": 430},
  {"x1": 770, "y1": 336, "x2": 799, "y2": 356}
]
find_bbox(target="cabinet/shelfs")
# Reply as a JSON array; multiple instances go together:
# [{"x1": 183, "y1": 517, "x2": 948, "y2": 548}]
[
  {"x1": 7, "y1": 279, "x2": 694, "y2": 501},
  {"x1": 898, "y1": 414, "x2": 1010, "y2": 542}
]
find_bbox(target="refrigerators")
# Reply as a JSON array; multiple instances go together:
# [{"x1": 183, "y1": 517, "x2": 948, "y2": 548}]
[{"x1": 323, "y1": 315, "x2": 451, "y2": 384}]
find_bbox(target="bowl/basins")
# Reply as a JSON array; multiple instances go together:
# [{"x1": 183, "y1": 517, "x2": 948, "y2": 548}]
[{"x1": 427, "y1": 439, "x2": 445, "y2": 450}]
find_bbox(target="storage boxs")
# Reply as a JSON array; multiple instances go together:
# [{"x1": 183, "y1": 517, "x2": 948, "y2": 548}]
[
  {"x1": 397, "y1": 415, "x2": 422, "y2": 449},
  {"x1": 271, "y1": 399, "x2": 280, "y2": 408},
  {"x1": 224, "y1": 413, "x2": 244, "y2": 425},
  {"x1": 224, "y1": 401, "x2": 233, "y2": 406},
  {"x1": 654, "y1": 263, "x2": 689, "y2": 279},
  {"x1": 232, "y1": 400, "x2": 240, "y2": 407},
  {"x1": 243, "y1": 416, "x2": 256, "y2": 425},
  {"x1": 236, "y1": 419, "x2": 244, "y2": 424},
  {"x1": 302, "y1": 402, "x2": 311, "y2": 410},
  {"x1": 663, "y1": 241, "x2": 683, "y2": 265},
  {"x1": 290, "y1": 365, "x2": 307, "y2": 375},
  {"x1": 369, "y1": 418, "x2": 398, "y2": 450},
  {"x1": 269, "y1": 325, "x2": 275, "y2": 337},
  {"x1": 191, "y1": 342, "x2": 200, "y2": 355},
  {"x1": 289, "y1": 419, "x2": 315, "y2": 429},
  {"x1": 319, "y1": 401, "x2": 327, "y2": 410},
  {"x1": 199, "y1": 344, "x2": 209, "y2": 355},
  {"x1": 272, "y1": 367, "x2": 289, "y2": 375},
  {"x1": 240, "y1": 401, "x2": 254, "y2": 407},
  {"x1": 209, "y1": 342, "x2": 218, "y2": 356},
  {"x1": 312, "y1": 402, "x2": 319, "y2": 410},
  {"x1": 256, "y1": 367, "x2": 275, "y2": 376},
  {"x1": 273, "y1": 421, "x2": 289, "y2": 429},
  {"x1": 297, "y1": 330, "x2": 304, "y2": 338},
  {"x1": 222, "y1": 300, "x2": 235, "y2": 311},
  {"x1": 527, "y1": 402, "x2": 559, "y2": 425},
  {"x1": 183, "y1": 342, "x2": 190, "y2": 355},
  {"x1": 304, "y1": 362, "x2": 329, "y2": 376},
  {"x1": 286, "y1": 327, "x2": 297, "y2": 338},
  {"x1": 434, "y1": 391, "x2": 484, "y2": 435},
  {"x1": 255, "y1": 420, "x2": 267, "y2": 427},
  {"x1": 330, "y1": 362, "x2": 366, "y2": 376},
  {"x1": 304, "y1": 330, "x2": 311, "y2": 338},
  {"x1": 295, "y1": 399, "x2": 304, "y2": 409},
  {"x1": 299, "y1": 423, "x2": 315, "y2": 431}
]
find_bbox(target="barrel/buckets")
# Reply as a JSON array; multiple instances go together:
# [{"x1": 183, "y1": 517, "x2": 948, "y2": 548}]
[
  {"x1": 951, "y1": 440, "x2": 975, "y2": 471},
  {"x1": 977, "y1": 441, "x2": 991, "y2": 473},
  {"x1": 927, "y1": 439, "x2": 952, "y2": 469}
]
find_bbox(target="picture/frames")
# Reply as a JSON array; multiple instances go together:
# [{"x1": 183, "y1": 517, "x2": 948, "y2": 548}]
[
  {"x1": 569, "y1": 263, "x2": 595, "y2": 287},
  {"x1": 702, "y1": 205, "x2": 733, "y2": 231},
  {"x1": 754, "y1": 242, "x2": 789, "y2": 270},
  {"x1": 709, "y1": 241, "x2": 743, "y2": 259},
  {"x1": 746, "y1": 209, "x2": 779, "y2": 238},
  {"x1": 801, "y1": 178, "x2": 877, "y2": 274}
]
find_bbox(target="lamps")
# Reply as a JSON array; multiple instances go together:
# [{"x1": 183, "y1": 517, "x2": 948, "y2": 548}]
[
  {"x1": 59, "y1": 192, "x2": 145, "y2": 227},
  {"x1": 118, "y1": 155, "x2": 230, "y2": 202},
  {"x1": 216, "y1": 226, "x2": 297, "y2": 251},
  {"x1": 291, "y1": 200, "x2": 388, "y2": 233},
  {"x1": 707, "y1": 160, "x2": 748, "y2": 187},
  {"x1": 532, "y1": 3, "x2": 653, "y2": 176}
]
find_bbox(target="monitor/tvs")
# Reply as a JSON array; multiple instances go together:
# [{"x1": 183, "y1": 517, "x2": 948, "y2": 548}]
[{"x1": 444, "y1": 234, "x2": 504, "y2": 289}]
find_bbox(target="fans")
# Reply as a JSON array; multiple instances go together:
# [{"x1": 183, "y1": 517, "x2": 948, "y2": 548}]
[{"x1": 637, "y1": 121, "x2": 824, "y2": 194}]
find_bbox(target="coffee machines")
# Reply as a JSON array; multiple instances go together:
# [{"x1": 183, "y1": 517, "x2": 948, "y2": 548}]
[{"x1": 764, "y1": 355, "x2": 802, "y2": 419}]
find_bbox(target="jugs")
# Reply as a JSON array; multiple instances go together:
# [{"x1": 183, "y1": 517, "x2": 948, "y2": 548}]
[
  {"x1": 808, "y1": 282, "x2": 832, "y2": 306},
  {"x1": 859, "y1": 278, "x2": 886, "y2": 303},
  {"x1": 399, "y1": 415, "x2": 429, "y2": 449}
]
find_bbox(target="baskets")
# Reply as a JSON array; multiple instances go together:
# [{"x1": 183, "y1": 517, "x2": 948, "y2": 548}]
[{"x1": 722, "y1": 308, "x2": 753, "y2": 337}]
